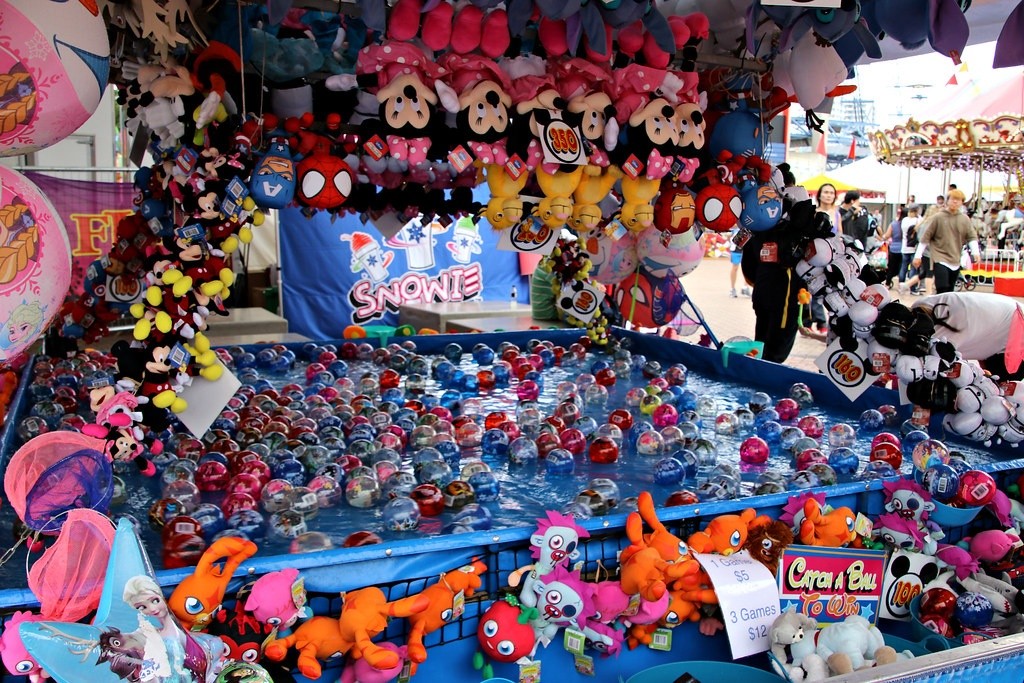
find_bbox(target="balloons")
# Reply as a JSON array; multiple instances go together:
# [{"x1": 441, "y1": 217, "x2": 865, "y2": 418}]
[
  {"x1": 0, "y1": 165, "x2": 71, "y2": 361},
  {"x1": 0, "y1": 0, "x2": 110, "y2": 158}
]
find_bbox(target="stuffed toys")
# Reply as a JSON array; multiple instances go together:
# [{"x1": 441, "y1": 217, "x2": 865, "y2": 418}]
[
  {"x1": 0, "y1": 467, "x2": 1024, "y2": 683},
  {"x1": 215, "y1": 0, "x2": 972, "y2": 235},
  {"x1": 789, "y1": 234, "x2": 1024, "y2": 447},
  {"x1": 46, "y1": 41, "x2": 264, "y2": 479},
  {"x1": 992, "y1": 0, "x2": 1024, "y2": 69},
  {"x1": 540, "y1": 235, "x2": 611, "y2": 345}
]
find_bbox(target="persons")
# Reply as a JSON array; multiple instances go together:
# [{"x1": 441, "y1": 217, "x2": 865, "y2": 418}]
[
  {"x1": 531, "y1": 228, "x2": 578, "y2": 321},
  {"x1": 731, "y1": 184, "x2": 1024, "y2": 381}
]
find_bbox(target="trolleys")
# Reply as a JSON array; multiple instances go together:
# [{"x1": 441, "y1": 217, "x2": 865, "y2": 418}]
[{"x1": 953, "y1": 243, "x2": 976, "y2": 291}]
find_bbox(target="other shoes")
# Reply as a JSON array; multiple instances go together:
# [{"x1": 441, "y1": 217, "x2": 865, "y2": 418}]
[
  {"x1": 729, "y1": 287, "x2": 752, "y2": 297},
  {"x1": 802, "y1": 327, "x2": 815, "y2": 338},
  {"x1": 818, "y1": 327, "x2": 828, "y2": 335},
  {"x1": 910, "y1": 285, "x2": 926, "y2": 296}
]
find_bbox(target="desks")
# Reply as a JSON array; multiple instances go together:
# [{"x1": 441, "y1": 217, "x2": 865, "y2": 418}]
[
  {"x1": 201, "y1": 307, "x2": 288, "y2": 337},
  {"x1": 446, "y1": 314, "x2": 579, "y2": 334},
  {"x1": 206, "y1": 332, "x2": 315, "y2": 348},
  {"x1": 399, "y1": 300, "x2": 533, "y2": 334}
]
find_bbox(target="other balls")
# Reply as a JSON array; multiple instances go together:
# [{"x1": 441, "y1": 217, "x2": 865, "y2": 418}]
[{"x1": 12, "y1": 335, "x2": 996, "y2": 573}]
